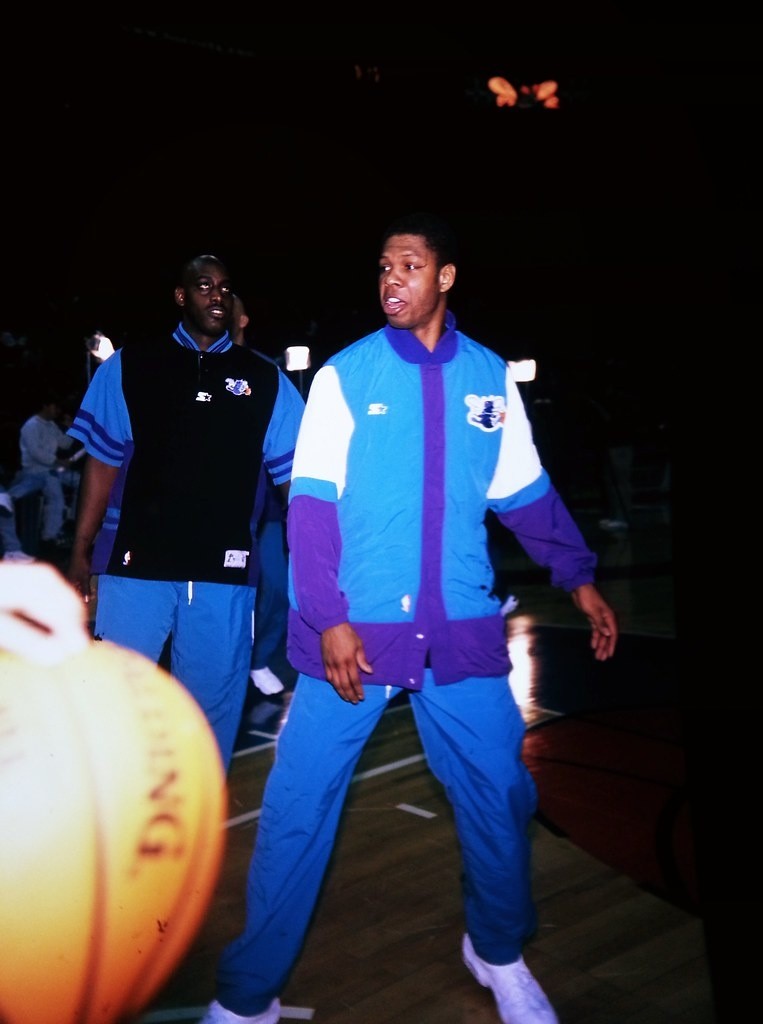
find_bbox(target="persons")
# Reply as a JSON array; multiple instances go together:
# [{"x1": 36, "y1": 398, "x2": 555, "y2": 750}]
[
  {"x1": 0, "y1": 397, "x2": 83, "y2": 564},
  {"x1": 64, "y1": 257, "x2": 306, "y2": 777},
  {"x1": 203, "y1": 222, "x2": 617, "y2": 1024}
]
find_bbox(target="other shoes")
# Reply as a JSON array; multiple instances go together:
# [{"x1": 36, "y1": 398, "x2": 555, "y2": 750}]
[
  {"x1": 2, "y1": 551, "x2": 35, "y2": 561},
  {"x1": 0, "y1": 493, "x2": 13, "y2": 516}
]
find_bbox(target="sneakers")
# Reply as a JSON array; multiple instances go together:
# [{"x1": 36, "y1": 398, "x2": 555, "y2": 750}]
[
  {"x1": 200, "y1": 997, "x2": 281, "y2": 1024},
  {"x1": 460, "y1": 934, "x2": 559, "y2": 1024},
  {"x1": 250, "y1": 666, "x2": 284, "y2": 695}
]
